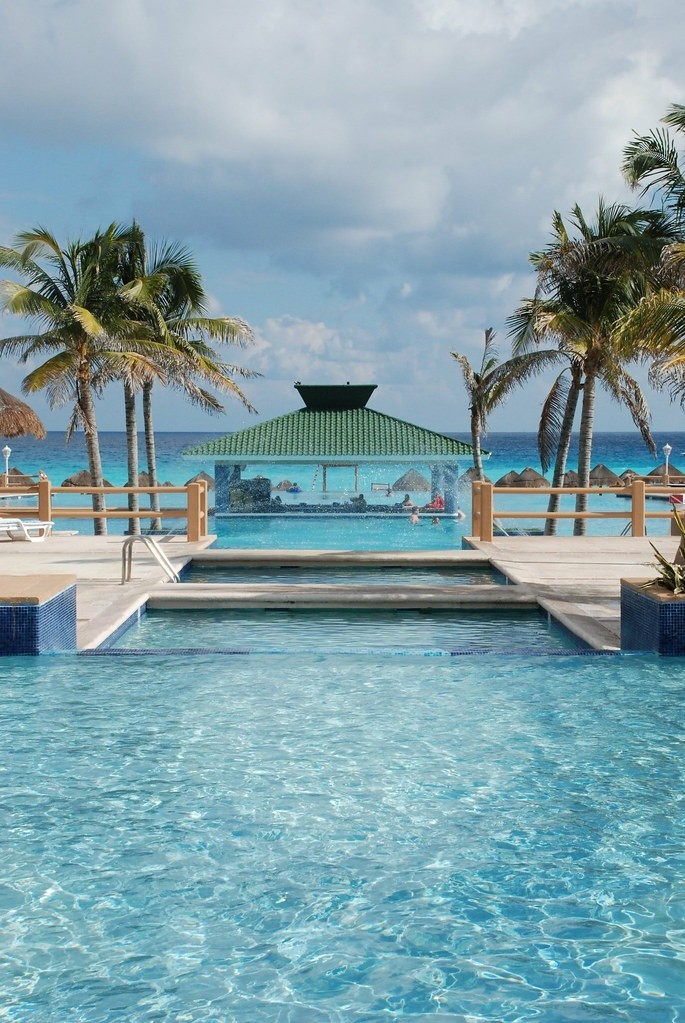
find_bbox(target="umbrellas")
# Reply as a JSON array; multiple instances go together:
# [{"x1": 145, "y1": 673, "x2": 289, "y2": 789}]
[
  {"x1": 392, "y1": 463, "x2": 685, "y2": 496},
  {"x1": 0, "y1": 388, "x2": 46, "y2": 440},
  {"x1": 0, "y1": 467, "x2": 293, "y2": 496}
]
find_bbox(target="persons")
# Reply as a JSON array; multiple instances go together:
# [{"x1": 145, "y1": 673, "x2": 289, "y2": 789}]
[
  {"x1": 424, "y1": 494, "x2": 445, "y2": 508},
  {"x1": 411, "y1": 506, "x2": 419, "y2": 523},
  {"x1": 386, "y1": 489, "x2": 393, "y2": 497},
  {"x1": 402, "y1": 494, "x2": 413, "y2": 506},
  {"x1": 432, "y1": 517, "x2": 439, "y2": 524},
  {"x1": 350, "y1": 494, "x2": 366, "y2": 512},
  {"x1": 458, "y1": 507, "x2": 464, "y2": 518},
  {"x1": 289, "y1": 482, "x2": 301, "y2": 491}
]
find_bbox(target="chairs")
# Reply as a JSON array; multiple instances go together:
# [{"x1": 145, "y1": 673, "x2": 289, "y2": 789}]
[{"x1": 0, "y1": 517, "x2": 54, "y2": 542}]
